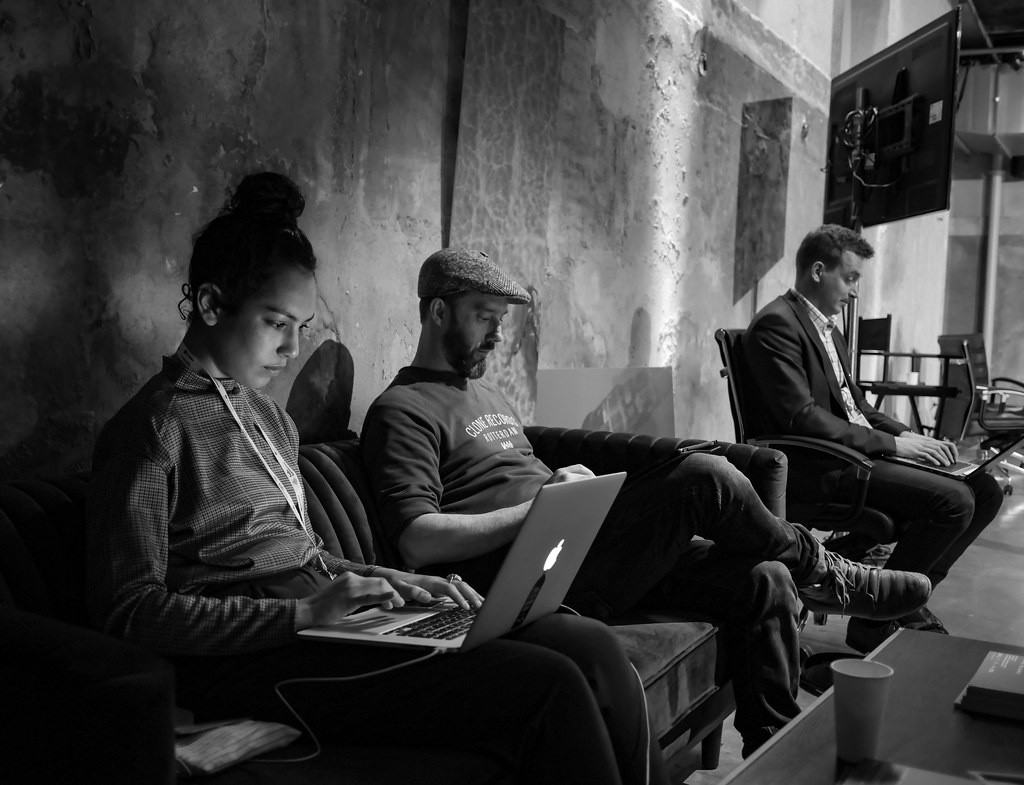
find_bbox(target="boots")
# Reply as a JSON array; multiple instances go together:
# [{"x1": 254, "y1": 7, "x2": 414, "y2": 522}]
[
  {"x1": 796, "y1": 533, "x2": 933, "y2": 619},
  {"x1": 740, "y1": 725, "x2": 780, "y2": 758}
]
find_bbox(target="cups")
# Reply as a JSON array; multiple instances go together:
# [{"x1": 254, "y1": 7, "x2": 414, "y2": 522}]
[
  {"x1": 830, "y1": 658, "x2": 894, "y2": 763},
  {"x1": 906, "y1": 371, "x2": 918, "y2": 385}
]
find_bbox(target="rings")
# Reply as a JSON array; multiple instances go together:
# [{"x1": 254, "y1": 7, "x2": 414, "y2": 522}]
[{"x1": 446, "y1": 573, "x2": 464, "y2": 585}]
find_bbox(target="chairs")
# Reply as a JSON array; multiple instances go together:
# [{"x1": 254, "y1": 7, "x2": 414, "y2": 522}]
[{"x1": 715, "y1": 315, "x2": 1024, "y2": 628}]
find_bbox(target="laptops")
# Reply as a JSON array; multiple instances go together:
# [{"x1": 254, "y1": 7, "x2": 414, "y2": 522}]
[
  {"x1": 881, "y1": 434, "x2": 1024, "y2": 481},
  {"x1": 296, "y1": 471, "x2": 627, "y2": 655}
]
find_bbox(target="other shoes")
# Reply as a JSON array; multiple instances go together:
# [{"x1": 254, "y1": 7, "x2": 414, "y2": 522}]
[{"x1": 846, "y1": 605, "x2": 949, "y2": 654}]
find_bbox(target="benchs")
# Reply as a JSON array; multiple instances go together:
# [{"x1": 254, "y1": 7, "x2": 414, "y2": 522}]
[{"x1": 0, "y1": 426, "x2": 788, "y2": 785}]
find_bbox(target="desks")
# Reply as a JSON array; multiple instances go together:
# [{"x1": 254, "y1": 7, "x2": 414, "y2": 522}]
[{"x1": 719, "y1": 627, "x2": 1024, "y2": 785}]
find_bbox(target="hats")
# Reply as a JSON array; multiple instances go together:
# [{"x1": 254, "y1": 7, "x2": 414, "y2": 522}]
[{"x1": 418, "y1": 248, "x2": 533, "y2": 305}]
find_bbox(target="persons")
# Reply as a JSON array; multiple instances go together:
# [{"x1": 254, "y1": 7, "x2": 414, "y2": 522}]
[
  {"x1": 346, "y1": 250, "x2": 933, "y2": 761},
  {"x1": 92, "y1": 170, "x2": 670, "y2": 785},
  {"x1": 736, "y1": 221, "x2": 1004, "y2": 653}
]
